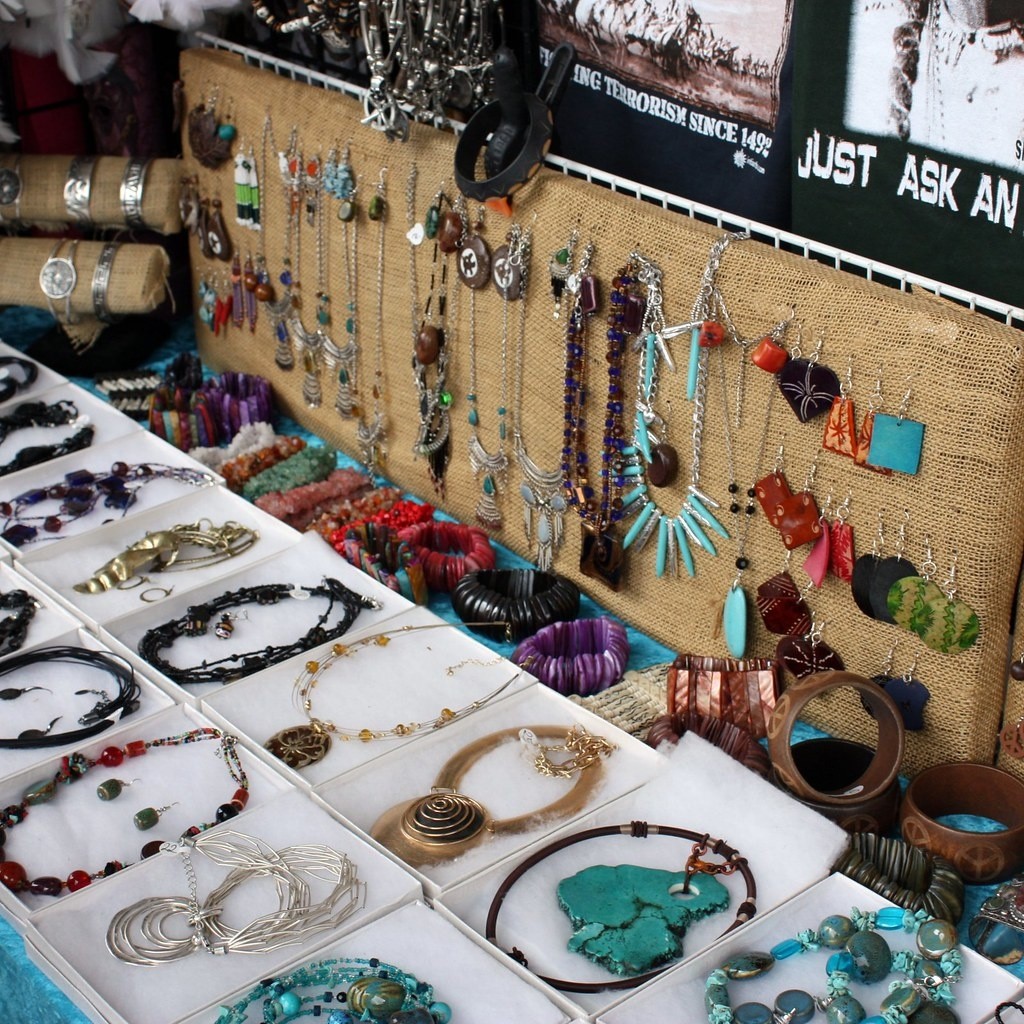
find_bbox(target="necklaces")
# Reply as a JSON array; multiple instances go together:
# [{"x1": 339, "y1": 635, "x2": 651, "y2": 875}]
[{"x1": 0, "y1": 1, "x2": 1023, "y2": 1024}]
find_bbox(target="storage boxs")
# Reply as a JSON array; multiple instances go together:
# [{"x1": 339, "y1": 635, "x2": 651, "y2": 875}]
[{"x1": 0, "y1": 340, "x2": 1023, "y2": 1024}]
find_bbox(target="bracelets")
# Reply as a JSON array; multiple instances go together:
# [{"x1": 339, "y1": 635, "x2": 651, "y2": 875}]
[
  {"x1": 344, "y1": 522, "x2": 427, "y2": 606},
  {"x1": 769, "y1": 670, "x2": 904, "y2": 806},
  {"x1": 903, "y1": 763, "x2": 1023, "y2": 887},
  {"x1": 120, "y1": 153, "x2": 151, "y2": 232},
  {"x1": 64, "y1": 153, "x2": 96, "y2": 224},
  {"x1": 455, "y1": 89, "x2": 553, "y2": 202},
  {"x1": 206, "y1": 372, "x2": 274, "y2": 434},
  {"x1": 91, "y1": 241, "x2": 121, "y2": 323},
  {"x1": 148, "y1": 384, "x2": 215, "y2": 452},
  {"x1": 398, "y1": 520, "x2": 493, "y2": 587},
  {"x1": 510, "y1": 615, "x2": 626, "y2": 696},
  {"x1": 38, "y1": 238, "x2": 82, "y2": 326},
  {"x1": 0, "y1": 151, "x2": 21, "y2": 223},
  {"x1": 452, "y1": 568, "x2": 581, "y2": 643}
]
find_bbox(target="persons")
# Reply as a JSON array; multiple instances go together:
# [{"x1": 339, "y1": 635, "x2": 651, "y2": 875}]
[{"x1": 842, "y1": 2, "x2": 1022, "y2": 172}]
